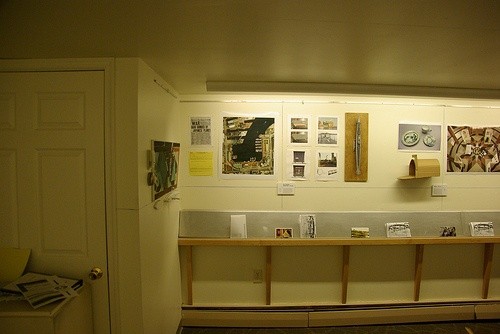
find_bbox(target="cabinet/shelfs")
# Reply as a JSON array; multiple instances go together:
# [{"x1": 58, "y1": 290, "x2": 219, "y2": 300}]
[{"x1": 0, "y1": 283, "x2": 93, "y2": 334}]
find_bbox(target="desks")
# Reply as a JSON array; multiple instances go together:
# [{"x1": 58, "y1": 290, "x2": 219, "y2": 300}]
[{"x1": 178, "y1": 237, "x2": 500, "y2": 305}]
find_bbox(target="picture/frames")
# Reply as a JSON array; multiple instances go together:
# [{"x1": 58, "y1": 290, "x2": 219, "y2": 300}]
[
  {"x1": 298, "y1": 214, "x2": 316, "y2": 239},
  {"x1": 151, "y1": 139, "x2": 181, "y2": 201},
  {"x1": 275, "y1": 228, "x2": 293, "y2": 239},
  {"x1": 218, "y1": 112, "x2": 278, "y2": 180}
]
